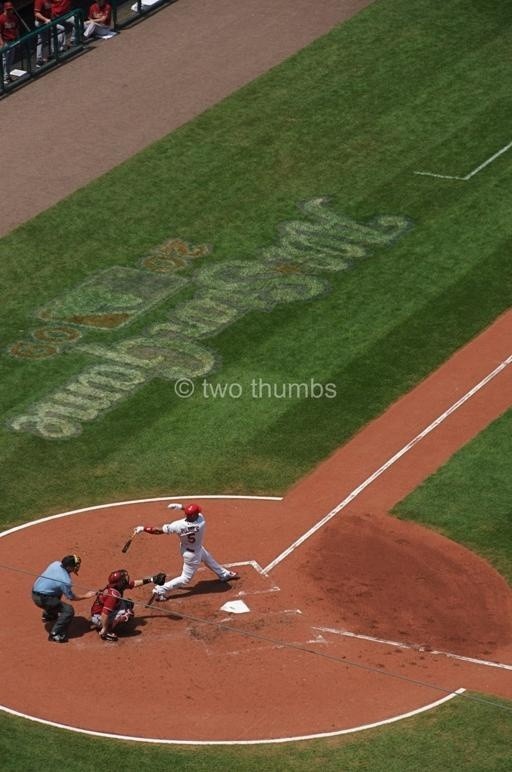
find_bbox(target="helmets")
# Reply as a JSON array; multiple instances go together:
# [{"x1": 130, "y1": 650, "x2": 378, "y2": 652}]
[
  {"x1": 183, "y1": 503, "x2": 202, "y2": 518},
  {"x1": 61, "y1": 553, "x2": 81, "y2": 567},
  {"x1": 108, "y1": 569, "x2": 130, "y2": 589}
]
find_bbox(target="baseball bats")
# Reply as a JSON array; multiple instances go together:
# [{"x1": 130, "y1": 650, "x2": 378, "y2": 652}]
[{"x1": 122, "y1": 530, "x2": 137, "y2": 553}]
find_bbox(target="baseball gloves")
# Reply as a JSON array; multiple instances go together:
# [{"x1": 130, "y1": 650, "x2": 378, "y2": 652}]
[{"x1": 153, "y1": 571, "x2": 166, "y2": 585}]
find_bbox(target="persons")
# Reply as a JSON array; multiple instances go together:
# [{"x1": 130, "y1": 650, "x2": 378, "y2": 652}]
[
  {"x1": 0, "y1": 2, "x2": 20, "y2": 86},
  {"x1": 66, "y1": 0, "x2": 114, "y2": 48},
  {"x1": 30, "y1": 553, "x2": 100, "y2": 644},
  {"x1": 89, "y1": 567, "x2": 166, "y2": 642},
  {"x1": 32, "y1": 0, "x2": 70, "y2": 69},
  {"x1": 131, "y1": 502, "x2": 240, "y2": 602}
]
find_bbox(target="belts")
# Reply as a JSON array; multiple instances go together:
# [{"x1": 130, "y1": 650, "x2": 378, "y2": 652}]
[{"x1": 31, "y1": 590, "x2": 56, "y2": 599}]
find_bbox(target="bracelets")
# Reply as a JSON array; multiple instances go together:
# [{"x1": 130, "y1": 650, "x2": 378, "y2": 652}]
[
  {"x1": 144, "y1": 526, "x2": 153, "y2": 535},
  {"x1": 177, "y1": 503, "x2": 182, "y2": 510},
  {"x1": 142, "y1": 578, "x2": 152, "y2": 586}
]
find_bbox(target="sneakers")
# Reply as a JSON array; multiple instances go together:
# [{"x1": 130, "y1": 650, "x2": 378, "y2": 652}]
[
  {"x1": 100, "y1": 631, "x2": 118, "y2": 641},
  {"x1": 41, "y1": 612, "x2": 68, "y2": 642},
  {"x1": 152, "y1": 588, "x2": 168, "y2": 601},
  {"x1": 219, "y1": 570, "x2": 238, "y2": 582}
]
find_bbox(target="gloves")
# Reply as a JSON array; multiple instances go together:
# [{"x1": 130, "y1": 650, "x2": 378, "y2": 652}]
[
  {"x1": 133, "y1": 525, "x2": 144, "y2": 534},
  {"x1": 168, "y1": 502, "x2": 183, "y2": 511}
]
